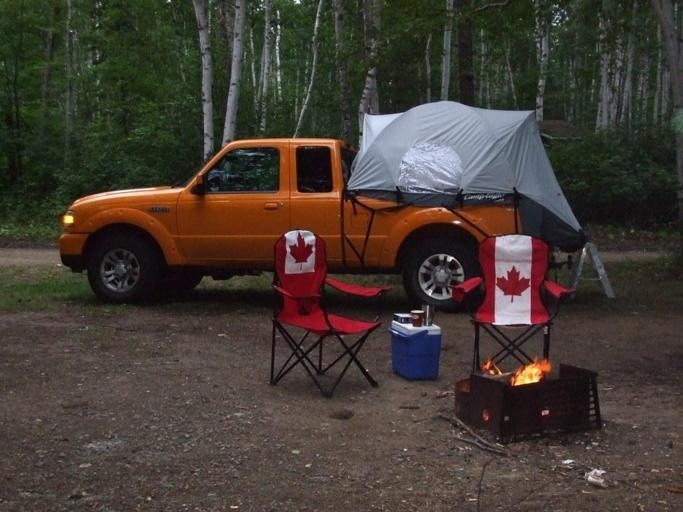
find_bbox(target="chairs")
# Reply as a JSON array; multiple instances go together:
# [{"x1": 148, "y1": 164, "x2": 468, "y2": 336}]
[
  {"x1": 268, "y1": 230, "x2": 393, "y2": 397},
  {"x1": 445, "y1": 235, "x2": 577, "y2": 379}
]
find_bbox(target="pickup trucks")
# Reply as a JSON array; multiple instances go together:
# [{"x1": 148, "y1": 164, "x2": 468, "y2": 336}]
[{"x1": 58, "y1": 137, "x2": 521, "y2": 313}]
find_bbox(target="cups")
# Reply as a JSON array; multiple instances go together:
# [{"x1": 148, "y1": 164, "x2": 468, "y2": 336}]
[
  {"x1": 411, "y1": 310, "x2": 424, "y2": 327},
  {"x1": 421, "y1": 304, "x2": 434, "y2": 327}
]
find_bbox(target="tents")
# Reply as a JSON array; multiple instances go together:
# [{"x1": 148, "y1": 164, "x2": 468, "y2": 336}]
[{"x1": 345, "y1": 99, "x2": 588, "y2": 254}]
